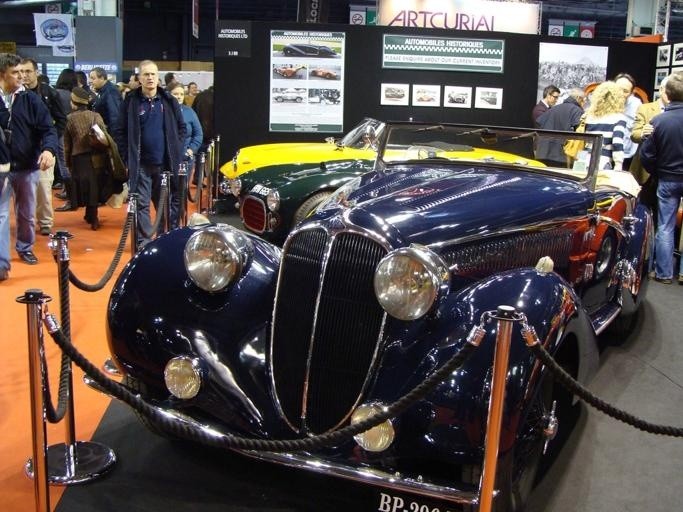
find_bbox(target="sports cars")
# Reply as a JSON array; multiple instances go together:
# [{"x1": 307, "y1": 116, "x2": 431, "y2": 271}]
[
  {"x1": 273, "y1": 91, "x2": 303, "y2": 103},
  {"x1": 234, "y1": 134, "x2": 534, "y2": 259},
  {"x1": 309, "y1": 67, "x2": 337, "y2": 80},
  {"x1": 273, "y1": 67, "x2": 296, "y2": 78},
  {"x1": 218, "y1": 115, "x2": 548, "y2": 216},
  {"x1": 282, "y1": 43, "x2": 337, "y2": 57},
  {"x1": 103, "y1": 120, "x2": 652, "y2": 512}
]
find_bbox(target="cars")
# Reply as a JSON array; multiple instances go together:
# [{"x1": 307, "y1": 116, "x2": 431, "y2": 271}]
[
  {"x1": 416, "y1": 93, "x2": 434, "y2": 102},
  {"x1": 455, "y1": 94, "x2": 466, "y2": 103},
  {"x1": 385, "y1": 88, "x2": 404, "y2": 98},
  {"x1": 44, "y1": 24, "x2": 65, "y2": 39}
]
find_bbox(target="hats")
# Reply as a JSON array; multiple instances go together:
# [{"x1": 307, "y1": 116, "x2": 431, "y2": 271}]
[{"x1": 70, "y1": 87, "x2": 88, "y2": 106}]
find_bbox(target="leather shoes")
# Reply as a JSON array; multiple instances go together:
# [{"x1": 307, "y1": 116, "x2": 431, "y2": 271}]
[{"x1": 1, "y1": 181, "x2": 100, "y2": 281}]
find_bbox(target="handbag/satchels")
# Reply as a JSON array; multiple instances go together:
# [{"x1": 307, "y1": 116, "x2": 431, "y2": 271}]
[
  {"x1": 88, "y1": 124, "x2": 110, "y2": 149},
  {"x1": 564, "y1": 121, "x2": 586, "y2": 158}
]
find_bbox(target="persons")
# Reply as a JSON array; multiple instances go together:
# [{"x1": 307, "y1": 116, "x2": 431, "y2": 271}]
[
  {"x1": 533, "y1": 73, "x2": 683, "y2": 284},
  {"x1": 0, "y1": 52, "x2": 214, "y2": 282}
]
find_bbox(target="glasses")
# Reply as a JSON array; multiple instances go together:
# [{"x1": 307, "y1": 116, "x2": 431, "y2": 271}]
[{"x1": 549, "y1": 94, "x2": 558, "y2": 96}]
